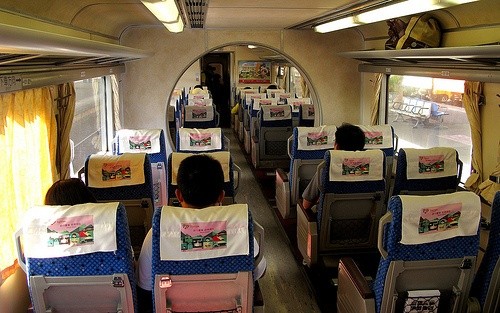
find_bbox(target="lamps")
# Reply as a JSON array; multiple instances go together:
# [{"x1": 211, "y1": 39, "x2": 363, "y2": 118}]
[
  {"x1": 140, "y1": 0, "x2": 185, "y2": 32},
  {"x1": 311, "y1": 0, "x2": 480, "y2": 33}
]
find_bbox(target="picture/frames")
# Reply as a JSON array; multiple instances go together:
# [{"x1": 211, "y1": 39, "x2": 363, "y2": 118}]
[{"x1": 238, "y1": 60, "x2": 272, "y2": 84}]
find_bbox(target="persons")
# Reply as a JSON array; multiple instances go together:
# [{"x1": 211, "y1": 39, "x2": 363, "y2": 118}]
[
  {"x1": 230, "y1": 86, "x2": 253, "y2": 115},
  {"x1": 44, "y1": 178, "x2": 98, "y2": 205},
  {"x1": 301, "y1": 122, "x2": 367, "y2": 209},
  {"x1": 134, "y1": 155, "x2": 267, "y2": 292}
]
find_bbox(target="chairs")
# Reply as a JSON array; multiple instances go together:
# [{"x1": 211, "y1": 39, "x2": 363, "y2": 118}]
[{"x1": 14, "y1": 86, "x2": 500, "y2": 313}]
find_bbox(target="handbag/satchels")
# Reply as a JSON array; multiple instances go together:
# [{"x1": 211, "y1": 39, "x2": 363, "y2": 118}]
[
  {"x1": 384, "y1": 18, "x2": 408, "y2": 49},
  {"x1": 396, "y1": 15, "x2": 442, "y2": 49}
]
